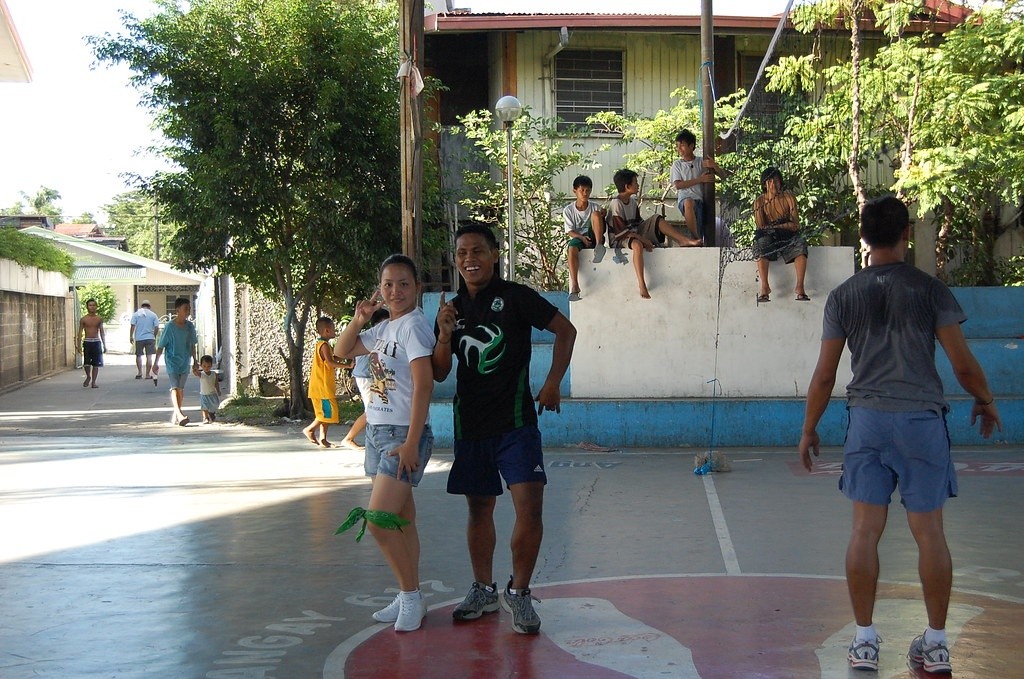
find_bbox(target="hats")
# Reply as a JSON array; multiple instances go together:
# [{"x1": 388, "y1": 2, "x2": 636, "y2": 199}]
[{"x1": 141, "y1": 300, "x2": 151, "y2": 306}]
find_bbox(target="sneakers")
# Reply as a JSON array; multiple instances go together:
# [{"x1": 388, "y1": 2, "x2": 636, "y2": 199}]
[
  {"x1": 453, "y1": 582, "x2": 501, "y2": 620},
  {"x1": 847, "y1": 636, "x2": 881, "y2": 671},
  {"x1": 909, "y1": 629, "x2": 952, "y2": 673},
  {"x1": 373, "y1": 590, "x2": 427, "y2": 632},
  {"x1": 501, "y1": 577, "x2": 542, "y2": 634}
]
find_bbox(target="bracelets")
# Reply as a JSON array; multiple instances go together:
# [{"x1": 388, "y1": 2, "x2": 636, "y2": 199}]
[
  {"x1": 438, "y1": 335, "x2": 451, "y2": 344},
  {"x1": 974, "y1": 398, "x2": 994, "y2": 405}
]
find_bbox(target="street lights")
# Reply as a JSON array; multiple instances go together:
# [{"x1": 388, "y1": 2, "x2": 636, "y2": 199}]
[{"x1": 495, "y1": 94, "x2": 521, "y2": 283}]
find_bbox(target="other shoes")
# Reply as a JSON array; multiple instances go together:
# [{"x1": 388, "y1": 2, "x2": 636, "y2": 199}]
[
  {"x1": 135, "y1": 374, "x2": 153, "y2": 379},
  {"x1": 172, "y1": 419, "x2": 190, "y2": 427},
  {"x1": 203, "y1": 418, "x2": 210, "y2": 424},
  {"x1": 83, "y1": 377, "x2": 98, "y2": 388}
]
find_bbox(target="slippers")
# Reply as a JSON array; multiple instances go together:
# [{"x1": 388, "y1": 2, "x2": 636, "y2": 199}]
[
  {"x1": 758, "y1": 295, "x2": 771, "y2": 302},
  {"x1": 568, "y1": 292, "x2": 583, "y2": 301},
  {"x1": 593, "y1": 243, "x2": 606, "y2": 263},
  {"x1": 795, "y1": 294, "x2": 811, "y2": 301}
]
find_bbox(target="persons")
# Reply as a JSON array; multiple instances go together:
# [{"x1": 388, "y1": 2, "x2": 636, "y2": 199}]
[
  {"x1": 130, "y1": 300, "x2": 159, "y2": 379},
  {"x1": 671, "y1": 129, "x2": 727, "y2": 245},
  {"x1": 333, "y1": 254, "x2": 436, "y2": 631},
  {"x1": 432, "y1": 223, "x2": 577, "y2": 634},
  {"x1": 77, "y1": 299, "x2": 106, "y2": 388},
  {"x1": 605, "y1": 169, "x2": 703, "y2": 299},
  {"x1": 152, "y1": 297, "x2": 199, "y2": 426},
  {"x1": 752, "y1": 167, "x2": 810, "y2": 301},
  {"x1": 193, "y1": 355, "x2": 222, "y2": 423},
  {"x1": 303, "y1": 317, "x2": 355, "y2": 448},
  {"x1": 798, "y1": 197, "x2": 1003, "y2": 672},
  {"x1": 563, "y1": 175, "x2": 607, "y2": 301}
]
